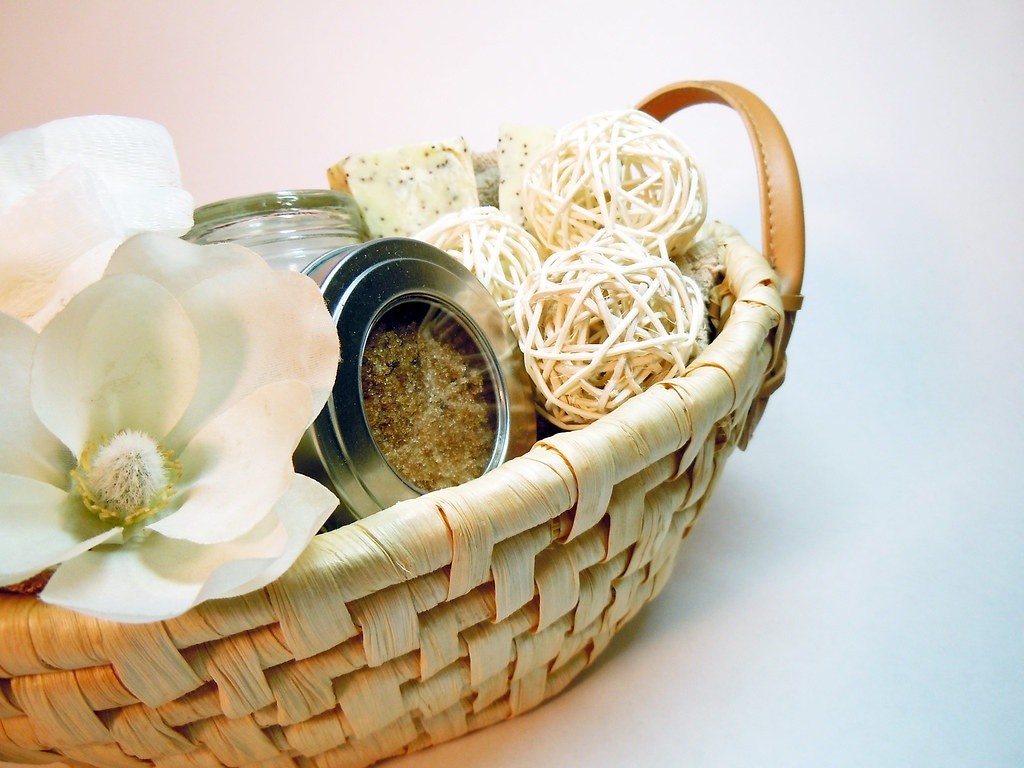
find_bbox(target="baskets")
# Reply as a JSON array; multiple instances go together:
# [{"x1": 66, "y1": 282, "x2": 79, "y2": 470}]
[{"x1": 0, "y1": 81, "x2": 805, "y2": 768}]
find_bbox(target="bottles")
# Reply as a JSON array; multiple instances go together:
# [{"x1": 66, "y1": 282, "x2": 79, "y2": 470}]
[{"x1": 181, "y1": 191, "x2": 364, "y2": 273}]
